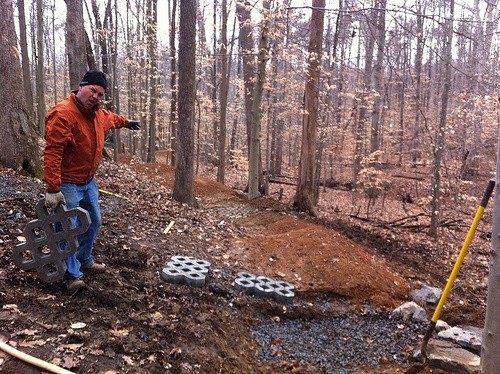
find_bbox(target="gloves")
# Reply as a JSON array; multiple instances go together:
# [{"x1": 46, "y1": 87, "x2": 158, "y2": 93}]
[
  {"x1": 44, "y1": 191, "x2": 66, "y2": 209},
  {"x1": 126, "y1": 119, "x2": 141, "y2": 130}
]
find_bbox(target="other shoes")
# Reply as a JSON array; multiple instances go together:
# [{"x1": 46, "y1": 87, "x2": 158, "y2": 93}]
[
  {"x1": 66, "y1": 276, "x2": 86, "y2": 293},
  {"x1": 79, "y1": 260, "x2": 107, "y2": 274}
]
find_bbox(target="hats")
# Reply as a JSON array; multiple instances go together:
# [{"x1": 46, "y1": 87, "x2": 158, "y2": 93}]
[{"x1": 79, "y1": 70, "x2": 108, "y2": 93}]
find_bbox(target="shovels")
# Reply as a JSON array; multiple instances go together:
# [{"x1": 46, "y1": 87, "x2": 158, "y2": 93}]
[{"x1": 404, "y1": 179, "x2": 496, "y2": 374}]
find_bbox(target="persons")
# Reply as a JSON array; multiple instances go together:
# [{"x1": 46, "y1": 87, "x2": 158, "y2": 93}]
[{"x1": 42, "y1": 69, "x2": 141, "y2": 292}]
